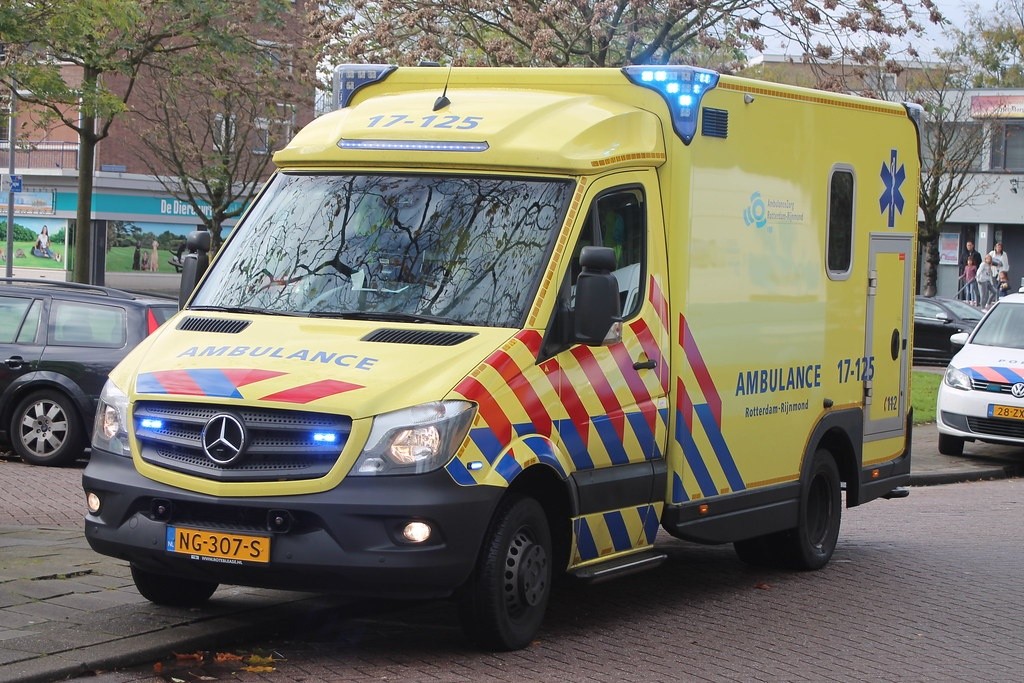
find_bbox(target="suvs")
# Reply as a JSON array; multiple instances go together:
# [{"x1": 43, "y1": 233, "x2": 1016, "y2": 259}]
[{"x1": 0, "y1": 276, "x2": 180, "y2": 467}]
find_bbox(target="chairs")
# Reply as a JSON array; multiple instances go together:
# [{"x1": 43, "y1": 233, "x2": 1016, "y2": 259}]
[{"x1": 62, "y1": 318, "x2": 92, "y2": 340}]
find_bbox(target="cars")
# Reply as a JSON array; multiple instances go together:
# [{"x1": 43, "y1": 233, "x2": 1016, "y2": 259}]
[
  {"x1": 913, "y1": 295, "x2": 986, "y2": 367},
  {"x1": 937, "y1": 286, "x2": 1024, "y2": 457}
]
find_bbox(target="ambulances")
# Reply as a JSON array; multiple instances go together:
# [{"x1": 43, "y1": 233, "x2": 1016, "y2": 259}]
[{"x1": 80, "y1": 63, "x2": 925, "y2": 655}]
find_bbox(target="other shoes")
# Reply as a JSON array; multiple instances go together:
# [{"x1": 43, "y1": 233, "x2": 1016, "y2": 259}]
[
  {"x1": 964, "y1": 300, "x2": 980, "y2": 307},
  {"x1": 985, "y1": 303, "x2": 993, "y2": 309},
  {"x1": 981, "y1": 309, "x2": 987, "y2": 314}
]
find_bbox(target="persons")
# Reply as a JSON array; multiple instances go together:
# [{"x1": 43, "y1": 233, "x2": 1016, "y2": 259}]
[
  {"x1": 33, "y1": 225, "x2": 56, "y2": 259},
  {"x1": 962, "y1": 241, "x2": 1012, "y2": 310}
]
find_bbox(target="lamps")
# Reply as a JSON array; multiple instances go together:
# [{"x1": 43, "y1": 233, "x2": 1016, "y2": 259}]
[{"x1": 1010, "y1": 179, "x2": 1024, "y2": 194}]
